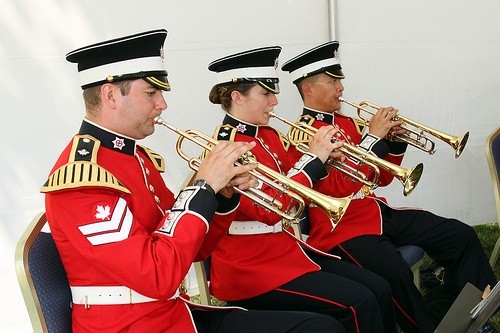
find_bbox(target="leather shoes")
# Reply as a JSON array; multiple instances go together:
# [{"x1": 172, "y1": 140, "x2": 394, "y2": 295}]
[{"x1": 478, "y1": 321, "x2": 494, "y2": 333}]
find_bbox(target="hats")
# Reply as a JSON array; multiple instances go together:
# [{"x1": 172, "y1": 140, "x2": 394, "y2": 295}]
[
  {"x1": 281, "y1": 40, "x2": 345, "y2": 84},
  {"x1": 65, "y1": 29, "x2": 170, "y2": 92},
  {"x1": 208, "y1": 45, "x2": 282, "y2": 94}
]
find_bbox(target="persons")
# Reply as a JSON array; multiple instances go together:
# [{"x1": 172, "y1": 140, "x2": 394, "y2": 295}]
[
  {"x1": 192, "y1": 47, "x2": 434, "y2": 333},
  {"x1": 40, "y1": 28, "x2": 351, "y2": 333},
  {"x1": 280, "y1": 38, "x2": 499, "y2": 333}
]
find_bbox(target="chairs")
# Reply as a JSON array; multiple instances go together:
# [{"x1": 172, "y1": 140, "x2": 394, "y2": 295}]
[
  {"x1": 289, "y1": 204, "x2": 426, "y2": 293},
  {"x1": 14, "y1": 211, "x2": 73, "y2": 333},
  {"x1": 484, "y1": 126, "x2": 500, "y2": 267},
  {"x1": 179, "y1": 161, "x2": 213, "y2": 306},
  {"x1": 40, "y1": 324, "x2": 43, "y2": 331}
]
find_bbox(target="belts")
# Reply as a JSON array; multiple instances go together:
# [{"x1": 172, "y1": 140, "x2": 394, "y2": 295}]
[
  {"x1": 228, "y1": 220, "x2": 282, "y2": 235},
  {"x1": 70, "y1": 285, "x2": 180, "y2": 305},
  {"x1": 309, "y1": 186, "x2": 369, "y2": 208}
]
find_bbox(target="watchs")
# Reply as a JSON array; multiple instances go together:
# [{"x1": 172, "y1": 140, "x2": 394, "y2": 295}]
[{"x1": 193, "y1": 178, "x2": 216, "y2": 195}]
